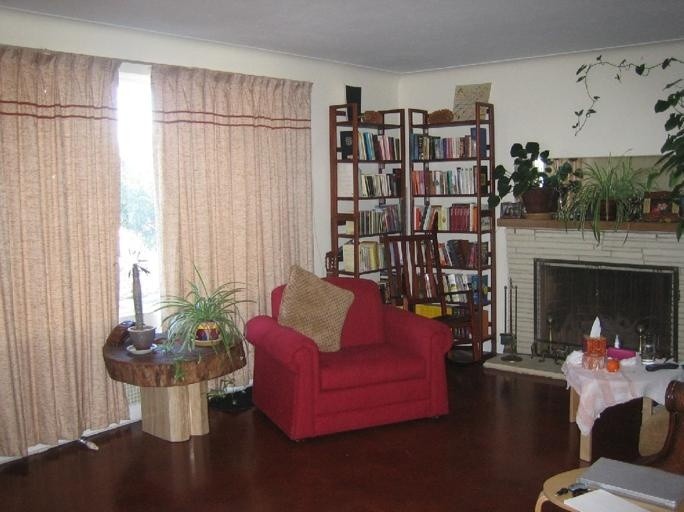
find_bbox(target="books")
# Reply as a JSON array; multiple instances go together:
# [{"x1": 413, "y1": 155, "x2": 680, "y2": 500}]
[
  {"x1": 564, "y1": 488, "x2": 648, "y2": 512},
  {"x1": 340, "y1": 127, "x2": 492, "y2": 339}
]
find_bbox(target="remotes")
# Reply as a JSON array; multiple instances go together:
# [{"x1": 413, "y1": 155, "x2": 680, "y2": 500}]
[{"x1": 646, "y1": 362, "x2": 679, "y2": 371}]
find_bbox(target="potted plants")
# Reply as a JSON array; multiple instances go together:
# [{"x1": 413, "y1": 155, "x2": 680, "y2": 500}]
[
  {"x1": 486, "y1": 143, "x2": 659, "y2": 244},
  {"x1": 126, "y1": 259, "x2": 257, "y2": 402}
]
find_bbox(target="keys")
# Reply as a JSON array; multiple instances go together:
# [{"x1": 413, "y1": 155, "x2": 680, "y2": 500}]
[{"x1": 555, "y1": 483, "x2": 592, "y2": 497}]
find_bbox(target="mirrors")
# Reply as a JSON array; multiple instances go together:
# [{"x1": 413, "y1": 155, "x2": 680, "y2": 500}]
[{"x1": 512, "y1": 155, "x2": 684, "y2": 214}]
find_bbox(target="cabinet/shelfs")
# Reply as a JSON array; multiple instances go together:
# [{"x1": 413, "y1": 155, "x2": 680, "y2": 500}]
[
  {"x1": 407, "y1": 102, "x2": 494, "y2": 360},
  {"x1": 329, "y1": 104, "x2": 406, "y2": 312}
]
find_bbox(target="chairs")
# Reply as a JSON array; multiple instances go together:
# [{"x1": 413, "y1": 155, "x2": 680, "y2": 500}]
[
  {"x1": 246, "y1": 276, "x2": 453, "y2": 444},
  {"x1": 379, "y1": 230, "x2": 480, "y2": 360}
]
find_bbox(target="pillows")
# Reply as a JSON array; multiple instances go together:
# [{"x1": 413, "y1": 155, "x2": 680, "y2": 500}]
[{"x1": 277, "y1": 264, "x2": 356, "y2": 352}]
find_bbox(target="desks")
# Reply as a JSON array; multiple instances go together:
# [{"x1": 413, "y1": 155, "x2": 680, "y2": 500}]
[
  {"x1": 102, "y1": 332, "x2": 245, "y2": 441},
  {"x1": 532, "y1": 350, "x2": 682, "y2": 511}
]
